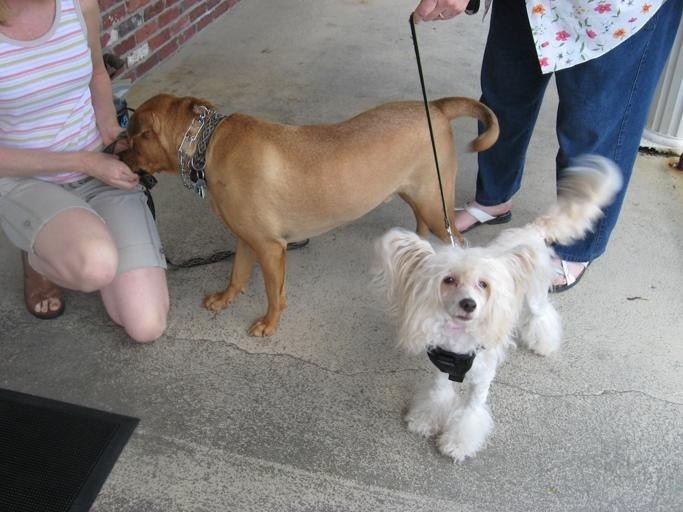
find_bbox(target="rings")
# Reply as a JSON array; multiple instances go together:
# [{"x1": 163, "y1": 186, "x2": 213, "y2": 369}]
[{"x1": 439, "y1": 13, "x2": 446, "y2": 20}]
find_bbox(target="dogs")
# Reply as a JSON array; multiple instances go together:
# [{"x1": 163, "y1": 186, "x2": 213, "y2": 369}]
[
  {"x1": 368, "y1": 155, "x2": 623, "y2": 463},
  {"x1": 101, "y1": 92, "x2": 500, "y2": 337}
]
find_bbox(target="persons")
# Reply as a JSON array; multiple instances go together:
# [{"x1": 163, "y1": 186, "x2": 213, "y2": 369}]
[
  {"x1": 0, "y1": 0, "x2": 170, "y2": 343},
  {"x1": 411, "y1": 0, "x2": 683, "y2": 295}
]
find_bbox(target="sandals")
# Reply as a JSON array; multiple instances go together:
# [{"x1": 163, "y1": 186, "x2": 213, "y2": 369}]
[
  {"x1": 21, "y1": 250, "x2": 65, "y2": 319},
  {"x1": 454, "y1": 201, "x2": 512, "y2": 234},
  {"x1": 548, "y1": 260, "x2": 592, "y2": 293}
]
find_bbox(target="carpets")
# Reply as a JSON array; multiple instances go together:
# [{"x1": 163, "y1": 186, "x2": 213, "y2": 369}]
[{"x1": 0, "y1": 386, "x2": 139, "y2": 511}]
